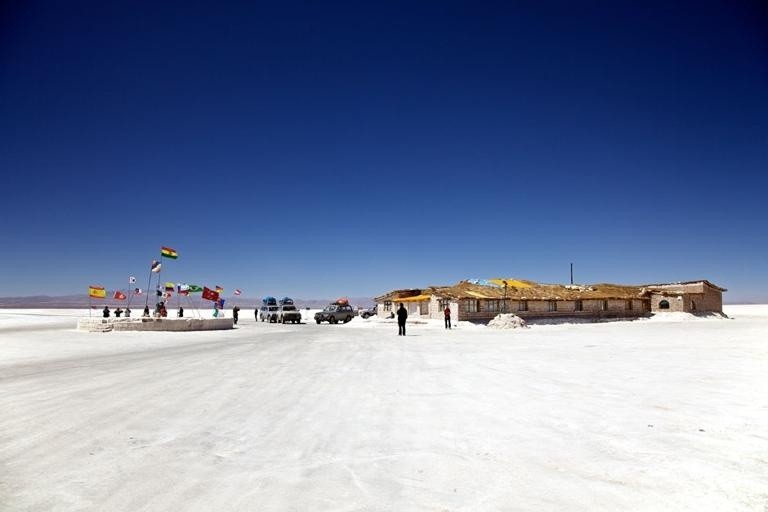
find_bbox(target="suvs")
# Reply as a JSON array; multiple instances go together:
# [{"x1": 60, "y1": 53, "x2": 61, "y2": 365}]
[
  {"x1": 313, "y1": 304, "x2": 354, "y2": 324},
  {"x1": 260, "y1": 303, "x2": 301, "y2": 324},
  {"x1": 359, "y1": 305, "x2": 378, "y2": 320}
]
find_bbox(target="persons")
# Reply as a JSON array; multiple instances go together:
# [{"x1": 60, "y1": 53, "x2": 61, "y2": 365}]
[
  {"x1": 113, "y1": 307, "x2": 123, "y2": 317},
  {"x1": 444, "y1": 305, "x2": 451, "y2": 328},
  {"x1": 144, "y1": 304, "x2": 149, "y2": 317},
  {"x1": 386, "y1": 311, "x2": 395, "y2": 319},
  {"x1": 177, "y1": 305, "x2": 184, "y2": 317},
  {"x1": 103, "y1": 306, "x2": 110, "y2": 317},
  {"x1": 124, "y1": 307, "x2": 132, "y2": 316},
  {"x1": 397, "y1": 303, "x2": 407, "y2": 336},
  {"x1": 233, "y1": 305, "x2": 240, "y2": 324},
  {"x1": 255, "y1": 308, "x2": 258, "y2": 321}
]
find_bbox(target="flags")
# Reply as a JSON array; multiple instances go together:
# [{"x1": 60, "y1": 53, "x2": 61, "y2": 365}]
[
  {"x1": 129, "y1": 276, "x2": 138, "y2": 284},
  {"x1": 133, "y1": 288, "x2": 142, "y2": 297},
  {"x1": 113, "y1": 290, "x2": 127, "y2": 300},
  {"x1": 151, "y1": 259, "x2": 162, "y2": 272},
  {"x1": 88, "y1": 285, "x2": 106, "y2": 298},
  {"x1": 156, "y1": 281, "x2": 225, "y2": 316},
  {"x1": 234, "y1": 288, "x2": 241, "y2": 296},
  {"x1": 160, "y1": 245, "x2": 177, "y2": 260}
]
